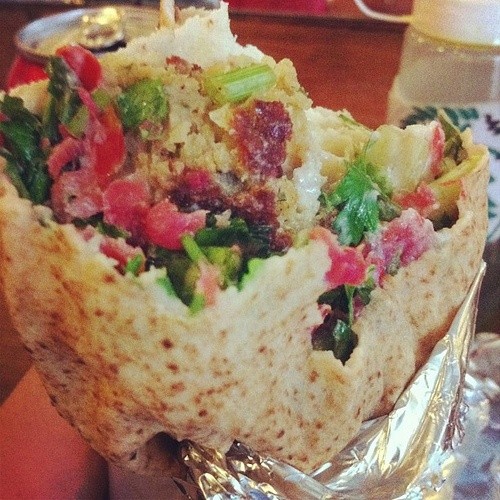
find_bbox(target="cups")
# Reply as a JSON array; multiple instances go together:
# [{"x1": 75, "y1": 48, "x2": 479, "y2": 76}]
[{"x1": 356, "y1": 3, "x2": 497, "y2": 250}]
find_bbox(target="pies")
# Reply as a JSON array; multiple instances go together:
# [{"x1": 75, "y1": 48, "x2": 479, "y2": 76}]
[{"x1": 0, "y1": 3, "x2": 491, "y2": 482}]
